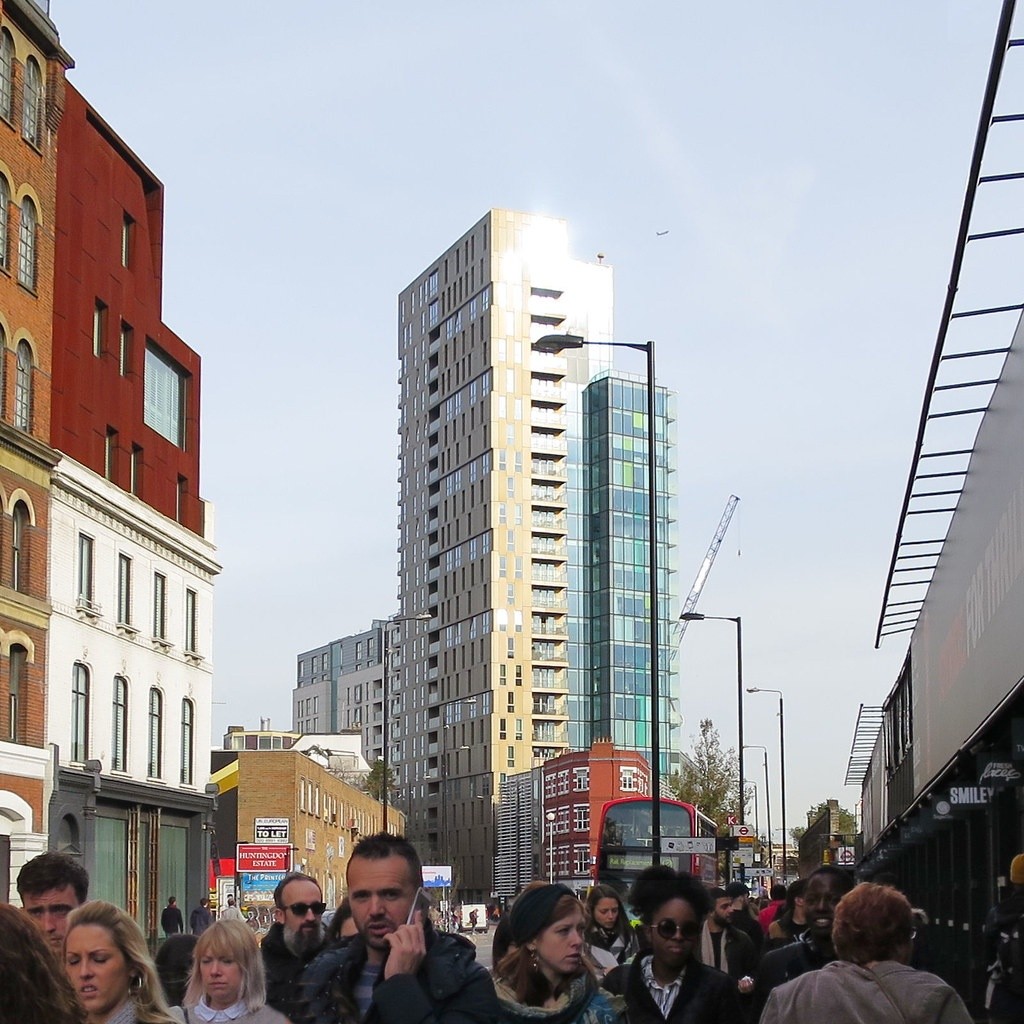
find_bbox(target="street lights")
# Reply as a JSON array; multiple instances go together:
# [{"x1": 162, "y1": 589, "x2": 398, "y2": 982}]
[
  {"x1": 746, "y1": 686, "x2": 788, "y2": 881},
  {"x1": 678, "y1": 611, "x2": 746, "y2": 887},
  {"x1": 546, "y1": 812, "x2": 555, "y2": 885},
  {"x1": 286, "y1": 847, "x2": 299, "y2": 879},
  {"x1": 442, "y1": 699, "x2": 479, "y2": 932},
  {"x1": 731, "y1": 779, "x2": 760, "y2": 884},
  {"x1": 382, "y1": 613, "x2": 431, "y2": 831},
  {"x1": 741, "y1": 744, "x2": 773, "y2": 889},
  {"x1": 531, "y1": 332, "x2": 660, "y2": 870}
]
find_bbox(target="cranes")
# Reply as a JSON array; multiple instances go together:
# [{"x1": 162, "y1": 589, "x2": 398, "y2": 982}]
[{"x1": 669, "y1": 492, "x2": 741, "y2": 662}]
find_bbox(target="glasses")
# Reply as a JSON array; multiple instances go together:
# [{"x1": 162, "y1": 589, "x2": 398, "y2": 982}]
[
  {"x1": 910, "y1": 924, "x2": 918, "y2": 941},
  {"x1": 280, "y1": 901, "x2": 326, "y2": 916},
  {"x1": 647, "y1": 919, "x2": 702, "y2": 942}
]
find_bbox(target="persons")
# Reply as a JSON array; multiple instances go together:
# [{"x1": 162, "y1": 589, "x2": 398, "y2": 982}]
[
  {"x1": 60, "y1": 898, "x2": 180, "y2": 1024},
  {"x1": 603, "y1": 822, "x2": 621, "y2": 846},
  {"x1": 488, "y1": 864, "x2": 759, "y2": 1024},
  {"x1": 265, "y1": 894, "x2": 359, "y2": 1014},
  {"x1": 162, "y1": 896, "x2": 184, "y2": 937},
  {"x1": 0, "y1": 903, "x2": 89, "y2": 1024},
  {"x1": 166, "y1": 917, "x2": 293, "y2": 1024},
  {"x1": 189, "y1": 898, "x2": 213, "y2": 937},
  {"x1": 17, "y1": 851, "x2": 90, "y2": 964},
  {"x1": 469, "y1": 909, "x2": 476, "y2": 935},
  {"x1": 267, "y1": 828, "x2": 505, "y2": 1024},
  {"x1": 753, "y1": 863, "x2": 976, "y2": 1024},
  {"x1": 258, "y1": 870, "x2": 328, "y2": 985},
  {"x1": 222, "y1": 898, "x2": 245, "y2": 921},
  {"x1": 982, "y1": 853, "x2": 1024, "y2": 1024}
]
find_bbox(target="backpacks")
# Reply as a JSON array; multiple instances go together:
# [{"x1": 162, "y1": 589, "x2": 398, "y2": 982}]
[{"x1": 998, "y1": 899, "x2": 1024, "y2": 994}]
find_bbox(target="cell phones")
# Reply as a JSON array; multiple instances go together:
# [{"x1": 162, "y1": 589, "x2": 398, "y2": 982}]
[{"x1": 407, "y1": 888, "x2": 432, "y2": 926}]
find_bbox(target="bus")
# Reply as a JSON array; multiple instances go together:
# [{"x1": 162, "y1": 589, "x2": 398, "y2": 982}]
[{"x1": 593, "y1": 796, "x2": 718, "y2": 889}]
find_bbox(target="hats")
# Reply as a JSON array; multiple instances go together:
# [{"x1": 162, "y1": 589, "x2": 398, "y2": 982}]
[
  {"x1": 726, "y1": 882, "x2": 758, "y2": 898},
  {"x1": 1010, "y1": 854, "x2": 1024, "y2": 884}
]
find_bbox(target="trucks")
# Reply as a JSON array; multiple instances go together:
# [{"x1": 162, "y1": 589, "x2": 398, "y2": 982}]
[{"x1": 458, "y1": 903, "x2": 489, "y2": 934}]
